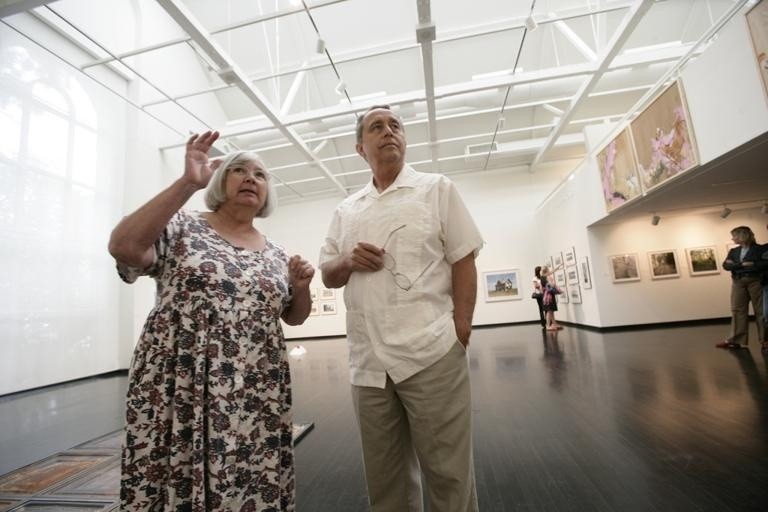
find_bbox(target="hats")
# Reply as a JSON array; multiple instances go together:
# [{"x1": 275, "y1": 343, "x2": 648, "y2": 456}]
[{"x1": 540, "y1": 265, "x2": 554, "y2": 278}]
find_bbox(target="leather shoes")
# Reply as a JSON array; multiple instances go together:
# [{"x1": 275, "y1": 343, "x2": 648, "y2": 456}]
[{"x1": 716, "y1": 339, "x2": 740, "y2": 348}]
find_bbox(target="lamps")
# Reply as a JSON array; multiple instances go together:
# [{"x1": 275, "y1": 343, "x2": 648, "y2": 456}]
[
  {"x1": 313, "y1": 12, "x2": 538, "y2": 134},
  {"x1": 651, "y1": 205, "x2": 733, "y2": 227}
]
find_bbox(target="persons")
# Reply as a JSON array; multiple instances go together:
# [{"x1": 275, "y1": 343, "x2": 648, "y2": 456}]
[
  {"x1": 717, "y1": 226, "x2": 767, "y2": 350},
  {"x1": 317, "y1": 102, "x2": 486, "y2": 511},
  {"x1": 531, "y1": 266, "x2": 552, "y2": 328},
  {"x1": 539, "y1": 261, "x2": 564, "y2": 331},
  {"x1": 107, "y1": 129, "x2": 317, "y2": 511}
]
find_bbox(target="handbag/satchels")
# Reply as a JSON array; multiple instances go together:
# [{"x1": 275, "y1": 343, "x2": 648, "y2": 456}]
[
  {"x1": 531, "y1": 287, "x2": 543, "y2": 299},
  {"x1": 546, "y1": 284, "x2": 560, "y2": 295}
]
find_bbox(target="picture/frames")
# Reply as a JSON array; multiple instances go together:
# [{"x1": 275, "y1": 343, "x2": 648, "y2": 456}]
[
  {"x1": 593, "y1": 76, "x2": 704, "y2": 215},
  {"x1": 483, "y1": 244, "x2": 593, "y2": 305},
  {"x1": 0, "y1": 422, "x2": 316, "y2": 512},
  {"x1": 308, "y1": 288, "x2": 337, "y2": 316},
  {"x1": 607, "y1": 243, "x2": 739, "y2": 283},
  {"x1": 741, "y1": 0, "x2": 767, "y2": 101}
]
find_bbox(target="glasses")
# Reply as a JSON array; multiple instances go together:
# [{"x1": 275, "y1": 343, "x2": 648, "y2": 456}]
[{"x1": 374, "y1": 223, "x2": 436, "y2": 295}]
